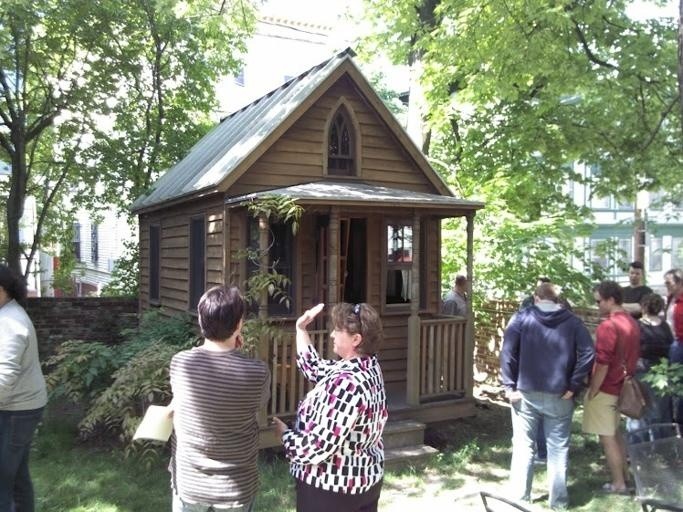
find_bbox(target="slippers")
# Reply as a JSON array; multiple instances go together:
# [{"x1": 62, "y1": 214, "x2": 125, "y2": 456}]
[{"x1": 592, "y1": 479, "x2": 632, "y2": 496}]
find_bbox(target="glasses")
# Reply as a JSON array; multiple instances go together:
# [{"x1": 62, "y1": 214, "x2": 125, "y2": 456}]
[{"x1": 596, "y1": 298, "x2": 602, "y2": 304}]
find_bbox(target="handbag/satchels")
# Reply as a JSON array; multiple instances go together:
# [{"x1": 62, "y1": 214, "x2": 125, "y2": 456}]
[{"x1": 618, "y1": 375, "x2": 652, "y2": 421}]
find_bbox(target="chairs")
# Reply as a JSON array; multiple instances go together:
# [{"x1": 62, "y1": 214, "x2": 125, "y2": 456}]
[{"x1": 621, "y1": 422, "x2": 683, "y2": 512}]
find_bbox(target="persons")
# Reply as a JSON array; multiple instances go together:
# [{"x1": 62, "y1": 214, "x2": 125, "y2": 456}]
[
  {"x1": 164, "y1": 284, "x2": 271, "y2": 512},
  {"x1": 0, "y1": 261, "x2": 48, "y2": 512},
  {"x1": 620, "y1": 261, "x2": 656, "y2": 322},
  {"x1": 623, "y1": 293, "x2": 675, "y2": 477},
  {"x1": 581, "y1": 280, "x2": 642, "y2": 494},
  {"x1": 442, "y1": 272, "x2": 480, "y2": 407},
  {"x1": 518, "y1": 277, "x2": 571, "y2": 463},
  {"x1": 662, "y1": 268, "x2": 682, "y2": 460},
  {"x1": 387, "y1": 247, "x2": 410, "y2": 303},
  {"x1": 499, "y1": 282, "x2": 595, "y2": 511},
  {"x1": 268, "y1": 301, "x2": 388, "y2": 511}
]
